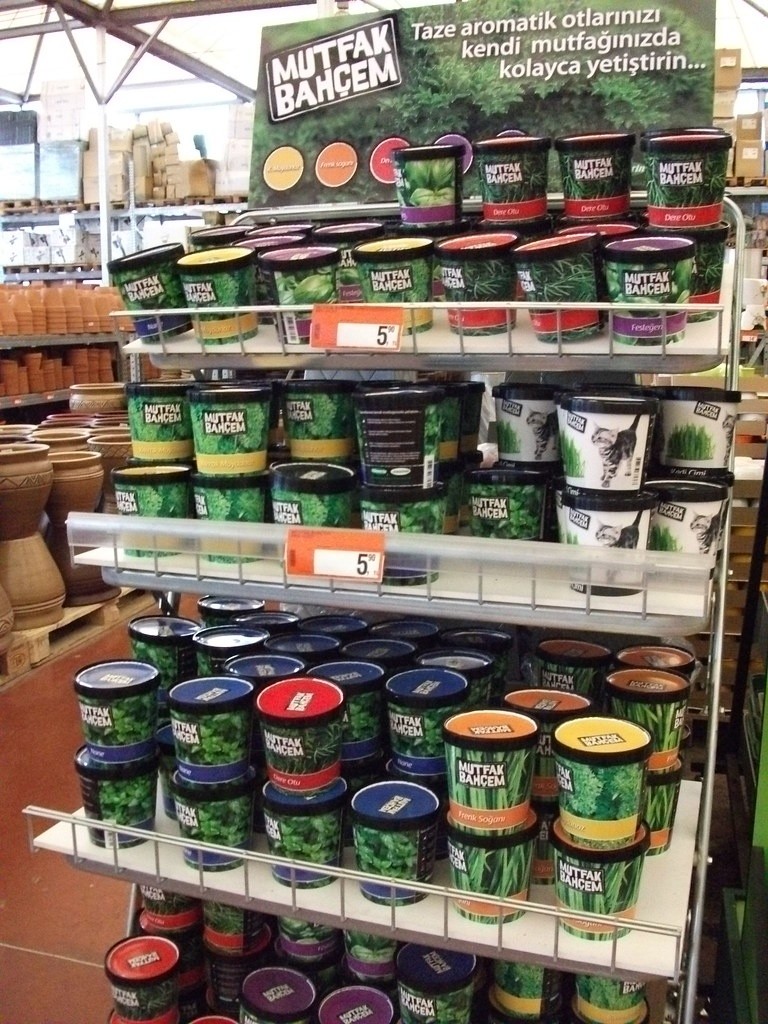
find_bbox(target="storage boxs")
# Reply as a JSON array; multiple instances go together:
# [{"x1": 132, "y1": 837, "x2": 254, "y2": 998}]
[
  {"x1": 0, "y1": 112, "x2": 220, "y2": 266},
  {"x1": 712, "y1": 47, "x2": 768, "y2": 179}
]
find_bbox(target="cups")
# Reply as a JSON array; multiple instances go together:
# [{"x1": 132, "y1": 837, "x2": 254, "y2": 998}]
[{"x1": 0, "y1": 122, "x2": 734, "y2": 1024}]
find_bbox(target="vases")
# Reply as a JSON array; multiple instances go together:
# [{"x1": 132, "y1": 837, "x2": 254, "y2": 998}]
[{"x1": 0, "y1": 285, "x2": 135, "y2": 636}]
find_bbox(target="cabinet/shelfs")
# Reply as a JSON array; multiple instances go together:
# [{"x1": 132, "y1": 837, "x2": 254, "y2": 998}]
[
  {"x1": 0, "y1": 200, "x2": 248, "y2": 282},
  {"x1": 22, "y1": 186, "x2": 768, "y2": 1024},
  {"x1": 0, "y1": 331, "x2": 131, "y2": 412}
]
